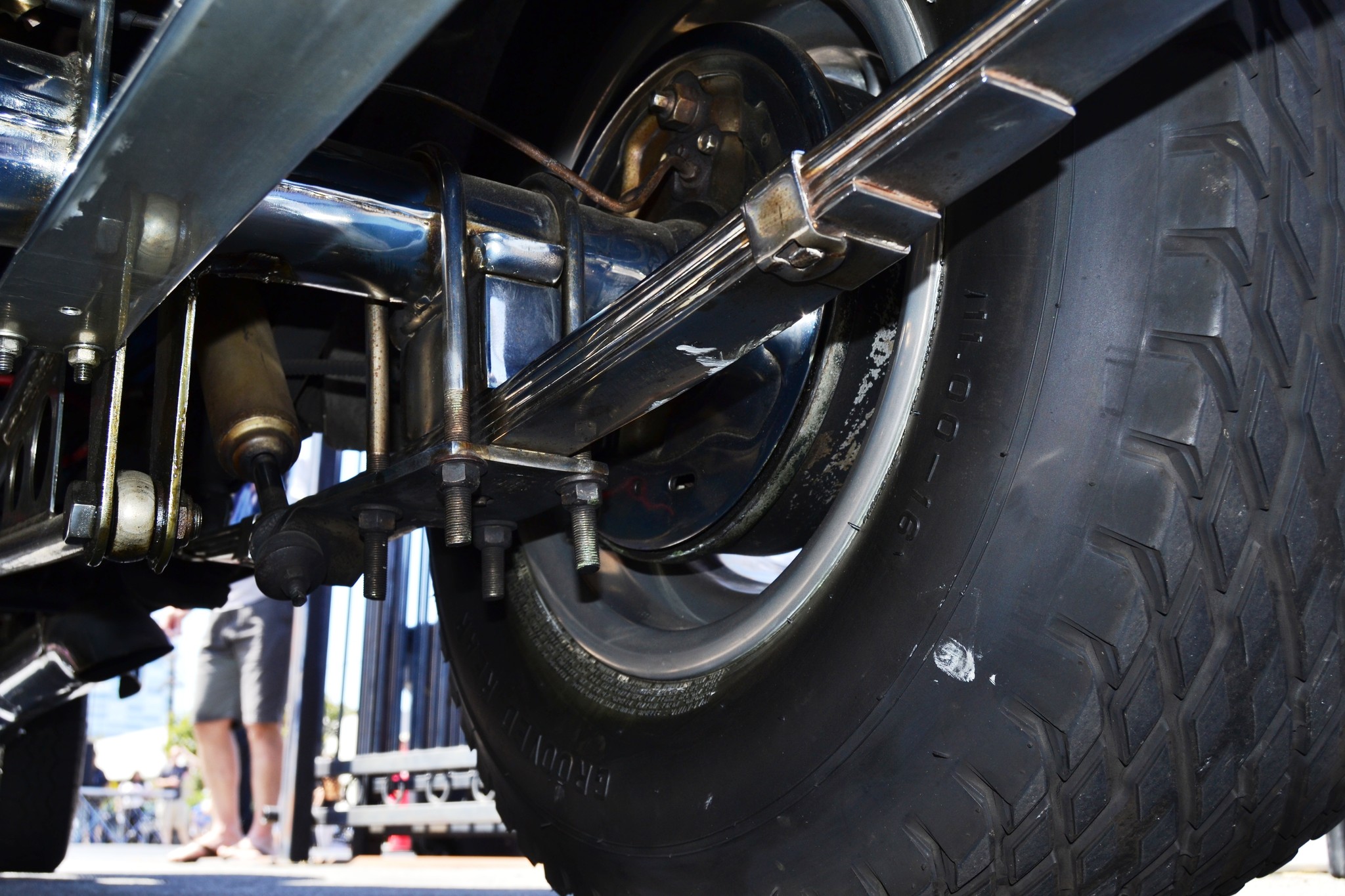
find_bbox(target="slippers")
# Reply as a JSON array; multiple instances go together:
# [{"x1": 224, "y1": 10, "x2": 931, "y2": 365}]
[{"x1": 167, "y1": 839, "x2": 274, "y2": 862}]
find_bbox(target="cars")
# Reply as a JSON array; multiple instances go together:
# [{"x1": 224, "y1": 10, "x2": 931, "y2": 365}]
[{"x1": 0, "y1": 0, "x2": 1345, "y2": 896}]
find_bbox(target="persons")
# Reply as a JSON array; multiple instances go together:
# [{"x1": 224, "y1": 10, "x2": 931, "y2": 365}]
[{"x1": 163, "y1": 475, "x2": 295, "y2": 863}]
